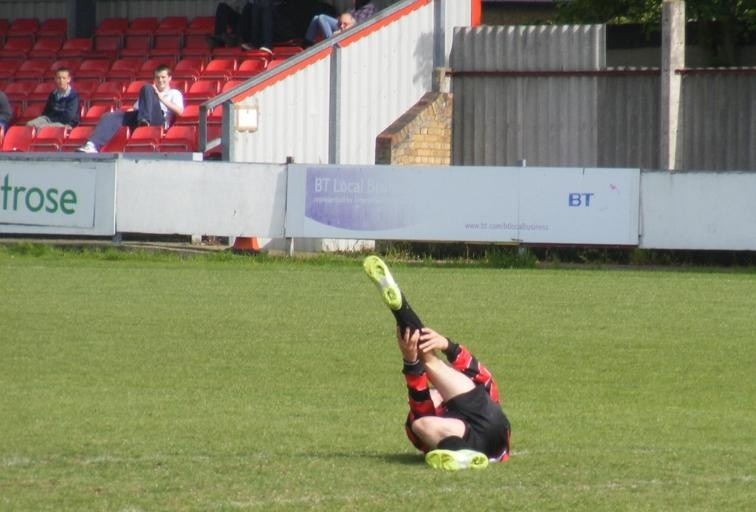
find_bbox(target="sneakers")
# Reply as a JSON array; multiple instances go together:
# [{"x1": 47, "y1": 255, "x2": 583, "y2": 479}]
[
  {"x1": 75, "y1": 141, "x2": 97, "y2": 153},
  {"x1": 363, "y1": 255, "x2": 404, "y2": 311},
  {"x1": 258, "y1": 41, "x2": 272, "y2": 54},
  {"x1": 241, "y1": 40, "x2": 256, "y2": 50},
  {"x1": 424, "y1": 448, "x2": 488, "y2": 472}
]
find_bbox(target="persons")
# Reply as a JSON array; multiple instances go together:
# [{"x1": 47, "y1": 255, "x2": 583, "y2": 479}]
[
  {"x1": 27, "y1": 68, "x2": 81, "y2": 133},
  {"x1": 363, "y1": 253, "x2": 515, "y2": 472},
  {"x1": 212, "y1": 1, "x2": 382, "y2": 53},
  {"x1": 74, "y1": 65, "x2": 184, "y2": 153}
]
